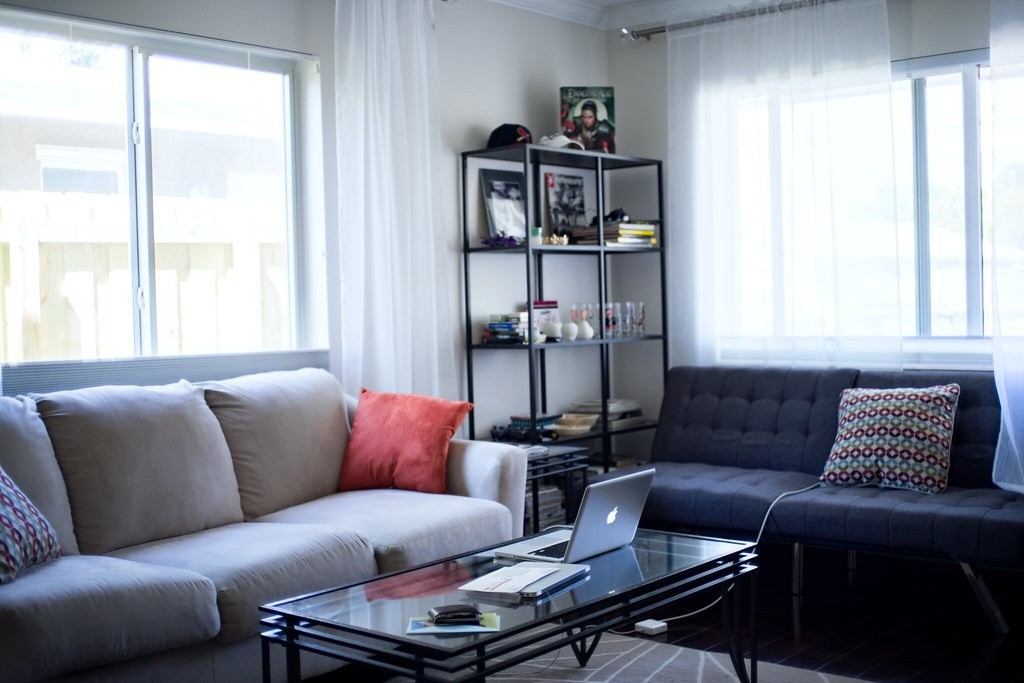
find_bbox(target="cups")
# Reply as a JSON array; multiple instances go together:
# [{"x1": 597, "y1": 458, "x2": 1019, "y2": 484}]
[{"x1": 571, "y1": 300, "x2": 645, "y2": 339}]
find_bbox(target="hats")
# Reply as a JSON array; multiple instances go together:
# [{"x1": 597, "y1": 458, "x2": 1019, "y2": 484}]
[
  {"x1": 538, "y1": 133, "x2": 585, "y2": 150},
  {"x1": 487, "y1": 123, "x2": 532, "y2": 148}
]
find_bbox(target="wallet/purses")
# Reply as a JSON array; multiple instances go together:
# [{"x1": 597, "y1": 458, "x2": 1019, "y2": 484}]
[{"x1": 428, "y1": 604, "x2": 483, "y2": 626}]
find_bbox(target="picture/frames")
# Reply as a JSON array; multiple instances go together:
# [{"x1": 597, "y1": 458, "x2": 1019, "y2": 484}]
[{"x1": 480, "y1": 168, "x2": 526, "y2": 245}]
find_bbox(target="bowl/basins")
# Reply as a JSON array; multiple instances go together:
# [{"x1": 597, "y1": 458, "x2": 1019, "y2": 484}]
[{"x1": 552, "y1": 412, "x2": 600, "y2": 435}]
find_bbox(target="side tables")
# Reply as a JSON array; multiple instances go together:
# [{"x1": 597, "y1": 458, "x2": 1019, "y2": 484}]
[{"x1": 504, "y1": 440, "x2": 589, "y2": 537}]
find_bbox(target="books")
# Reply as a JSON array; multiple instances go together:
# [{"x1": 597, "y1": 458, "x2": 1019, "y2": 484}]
[
  {"x1": 479, "y1": 312, "x2": 548, "y2": 344},
  {"x1": 569, "y1": 398, "x2": 646, "y2": 430},
  {"x1": 524, "y1": 483, "x2": 566, "y2": 534},
  {"x1": 572, "y1": 218, "x2": 660, "y2": 248},
  {"x1": 574, "y1": 451, "x2": 648, "y2": 478}
]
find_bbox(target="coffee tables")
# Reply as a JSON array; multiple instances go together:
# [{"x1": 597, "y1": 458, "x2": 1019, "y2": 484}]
[{"x1": 257, "y1": 526, "x2": 761, "y2": 683}]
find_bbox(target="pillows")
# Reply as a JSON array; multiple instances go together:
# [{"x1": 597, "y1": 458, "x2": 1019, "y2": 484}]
[
  {"x1": 337, "y1": 386, "x2": 474, "y2": 494},
  {"x1": 818, "y1": 383, "x2": 960, "y2": 496},
  {"x1": 0, "y1": 465, "x2": 64, "y2": 585},
  {"x1": 0, "y1": 394, "x2": 81, "y2": 557},
  {"x1": 25, "y1": 378, "x2": 245, "y2": 556},
  {"x1": 191, "y1": 366, "x2": 350, "y2": 522}
]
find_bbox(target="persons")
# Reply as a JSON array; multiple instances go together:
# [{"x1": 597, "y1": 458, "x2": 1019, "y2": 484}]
[
  {"x1": 489, "y1": 181, "x2": 527, "y2": 237},
  {"x1": 570, "y1": 101, "x2": 615, "y2": 154}
]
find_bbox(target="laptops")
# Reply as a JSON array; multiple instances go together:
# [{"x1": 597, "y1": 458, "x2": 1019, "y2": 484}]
[{"x1": 495, "y1": 467, "x2": 656, "y2": 564}]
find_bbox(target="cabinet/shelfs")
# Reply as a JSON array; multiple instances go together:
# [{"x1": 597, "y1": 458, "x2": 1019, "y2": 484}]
[{"x1": 460, "y1": 142, "x2": 669, "y2": 486}]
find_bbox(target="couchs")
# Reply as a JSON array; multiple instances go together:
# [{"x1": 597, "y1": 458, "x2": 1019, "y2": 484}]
[
  {"x1": 0, "y1": 366, "x2": 528, "y2": 683},
  {"x1": 567, "y1": 366, "x2": 1024, "y2": 635}
]
